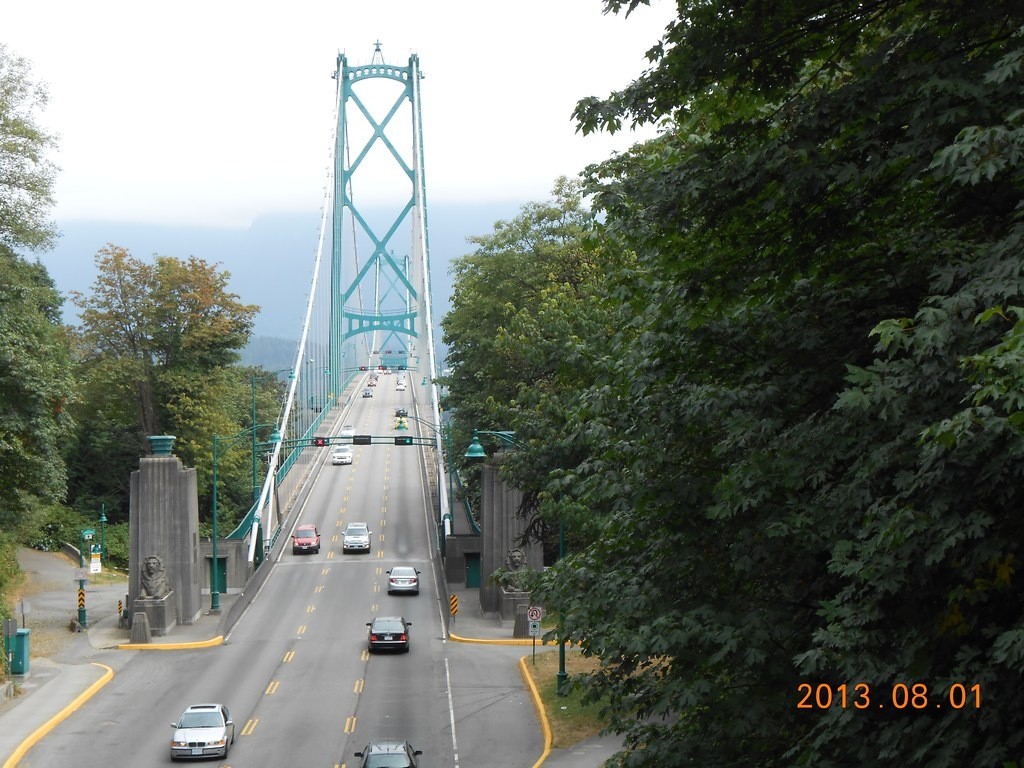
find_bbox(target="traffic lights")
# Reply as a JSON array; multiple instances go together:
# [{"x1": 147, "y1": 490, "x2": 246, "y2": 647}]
[
  {"x1": 315, "y1": 438, "x2": 323, "y2": 448},
  {"x1": 373, "y1": 351, "x2": 404, "y2": 354},
  {"x1": 358, "y1": 366, "x2": 406, "y2": 371},
  {"x1": 395, "y1": 437, "x2": 413, "y2": 446}
]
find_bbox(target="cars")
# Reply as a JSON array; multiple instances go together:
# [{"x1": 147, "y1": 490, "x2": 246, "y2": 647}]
[
  {"x1": 331, "y1": 445, "x2": 354, "y2": 466},
  {"x1": 393, "y1": 416, "x2": 409, "y2": 430},
  {"x1": 339, "y1": 425, "x2": 357, "y2": 438},
  {"x1": 291, "y1": 525, "x2": 321, "y2": 555},
  {"x1": 394, "y1": 406, "x2": 408, "y2": 417},
  {"x1": 365, "y1": 616, "x2": 412, "y2": 656},
  {"x1": 360, "y1": 368, "x2": 408, "y2": 398},
  {"x1": 170, "y1": 704, "x2": 235, "y2": 760},
  {"x1": 386, "y1": 567, "x2": 421, "y2": 597}
]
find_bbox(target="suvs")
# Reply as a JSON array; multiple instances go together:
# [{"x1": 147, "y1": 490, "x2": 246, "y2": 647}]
[
  {"x1": 342, "y1": 522, "x2": 373, "y2": 553},
  {"x1": 354, "y1": 739, "x2": 423, "y2": 768}
]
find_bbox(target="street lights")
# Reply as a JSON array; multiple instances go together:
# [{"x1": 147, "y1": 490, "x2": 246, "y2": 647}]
[
  {"x1": 78, "y1": 512, "x2": 108, "y2": 626},
  {"x1": 210, "y1": 423, "x2": 281, "y2": 613},
  {"x1": 464, "y1": 429, "x2": 570, "y2": 696},
  {"x1": 394, "y1": 415, "x2": 455, "y2": 536},
  {"x1": 251, "y1": 366, "x2": 296, "y2": 569}
]
